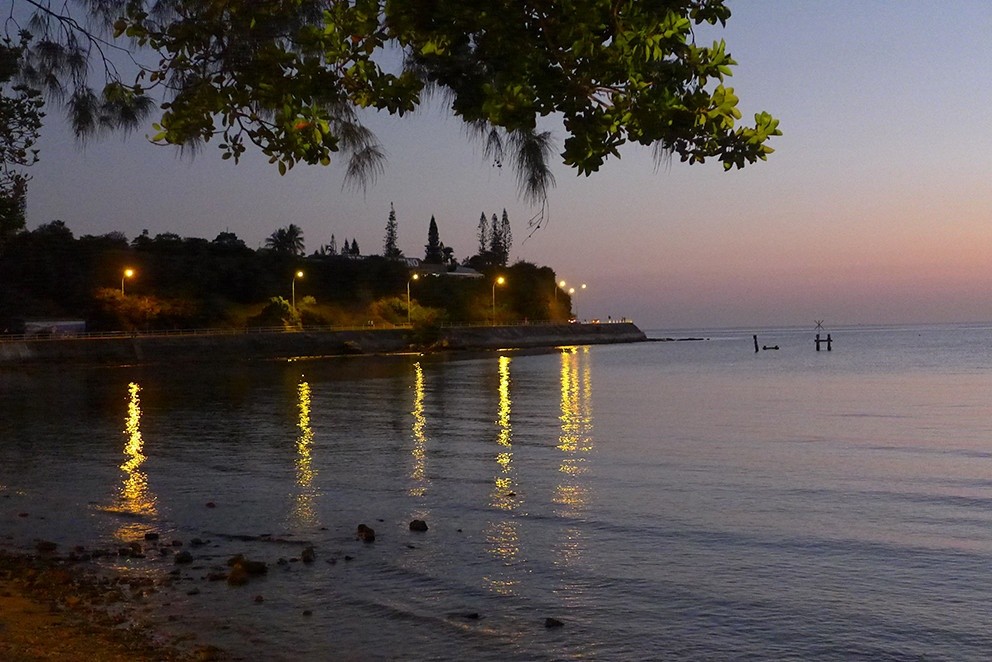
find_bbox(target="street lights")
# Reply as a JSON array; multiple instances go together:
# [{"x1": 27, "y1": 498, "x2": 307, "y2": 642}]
[
  {"x1": 554, "y1": 280, "x2": 566, "y2": 299},
  {"x1": 120, "y1": 268, "x2": 134, "y2": 297},
  {"x1": 492, "y1": 277, "x2": 504, "y2": 314},
  {"x1": 292, "y1": 271, "x2": 304, "y2": 308},
  {"x1": 407, "y1": 274, "x2": 418, "y2": 321}
]
